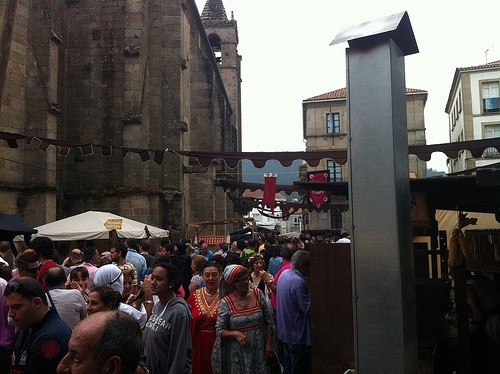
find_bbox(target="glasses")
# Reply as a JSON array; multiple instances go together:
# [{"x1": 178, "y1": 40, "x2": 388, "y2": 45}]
[
  {"x1": 22, "y1": 264, "x2": 41, "y2": 273},
  {"x1": 236, "y1": 275, "x2": 249, "y2": 284}
]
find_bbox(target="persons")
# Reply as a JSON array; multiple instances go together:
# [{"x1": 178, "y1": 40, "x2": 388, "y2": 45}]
[{"x1": 0, "y1": 224, "x2": 351, "y2": 374}]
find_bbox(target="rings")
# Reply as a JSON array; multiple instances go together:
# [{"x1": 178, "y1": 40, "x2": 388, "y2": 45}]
[{"x1": 243, "y1": 341, "x2": 244, "y2": 343}]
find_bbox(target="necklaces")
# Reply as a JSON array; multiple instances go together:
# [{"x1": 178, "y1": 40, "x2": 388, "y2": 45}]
[{"x1": 233, "y1": 292, "x2": 246, "y2": 300}]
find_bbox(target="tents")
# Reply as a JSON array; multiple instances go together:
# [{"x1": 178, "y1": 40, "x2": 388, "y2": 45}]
[{"x1": 14, "y1": 211, "x2": 169, "y2": 252}]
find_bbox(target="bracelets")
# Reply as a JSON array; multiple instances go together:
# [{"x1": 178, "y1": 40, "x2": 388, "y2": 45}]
[{"x1": 145, "y1": 300, "x2": 153, "y2": 303}]
